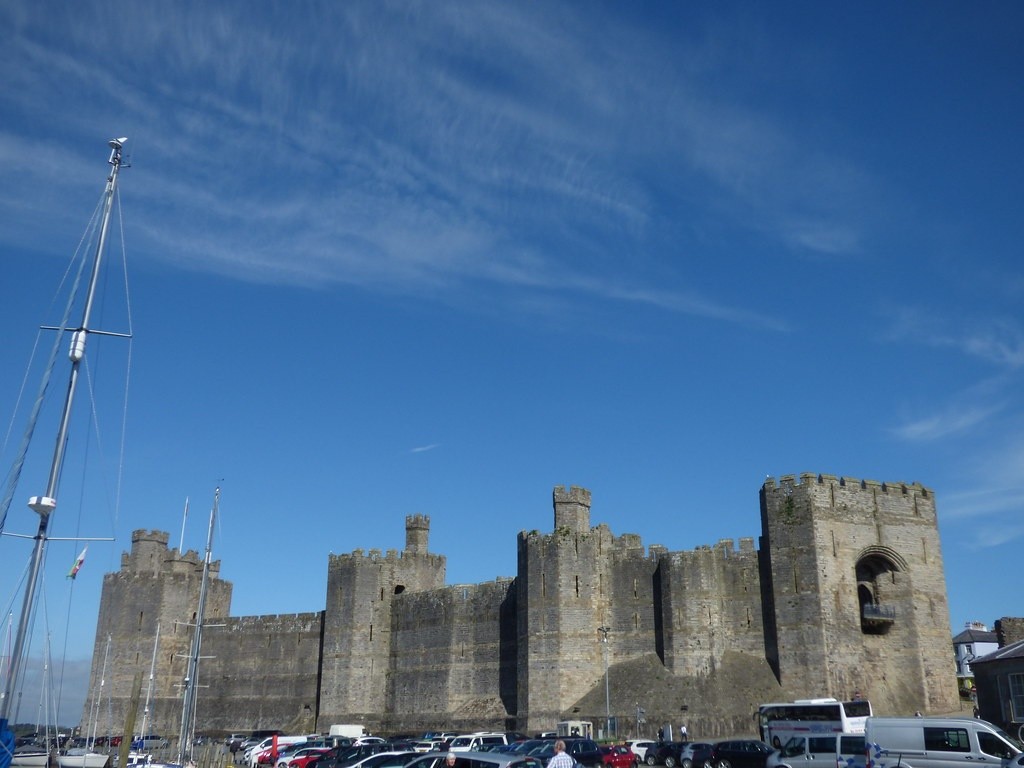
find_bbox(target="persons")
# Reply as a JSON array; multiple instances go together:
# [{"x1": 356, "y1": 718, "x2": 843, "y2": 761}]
[
  {"x1": 657, "y1": 726, "x2": 664, "y2": 741},
  {"x1": 230, "y1": 740, "x2": 239, "y2": 760},
  {"x1": 973, "y1": 705, "x2": 980, "y2": 719},
  {"x1": 547, "y1": 740, "x2": 573, "y2": 768},
  {"x1": 443, "y1": 752, "x2": 456, "y2": 768},
  {"x1": 681, "y1": 724, "x2": 687, "y2": 742}
]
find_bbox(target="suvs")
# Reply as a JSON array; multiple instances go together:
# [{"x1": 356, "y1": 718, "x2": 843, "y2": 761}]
[
  {"x1": 241, "y1": 730, "x2": 284, "y2": 748},
  {"x1": 491, "y1": 736, "x2": 604, "y2": 768}
]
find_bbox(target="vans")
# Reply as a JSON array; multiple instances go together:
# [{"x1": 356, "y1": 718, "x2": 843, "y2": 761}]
[
  {"x1": 250, "y1": 736, "x2": 307, "y2": 761},
  {"x1": 330, "y1": 725, "x2": 369, "y2": 738},
  {"x1": 138, "y1": 736, "x2": 168, "y2": 749},
  {"x1": 624, "y1": 739, "x2": 658, "y2": 763},
  {"x1": 450, "y1": 732, "x2": 508, "y2": 751},
  {"x1": 864, "y1": 716, "x2": 1024, "y2": 768},
  {"x1": 226, "y1": 734, "x2": 247, "y2": 746},
  {"x1": 778, "y1": 734, "x2": 868, "y2": 768},
  {"x1": 709, "y1": 739, "x2": 776, "y2": 768}
]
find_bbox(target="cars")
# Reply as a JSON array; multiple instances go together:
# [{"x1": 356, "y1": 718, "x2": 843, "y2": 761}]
[
  {"x1": 273, "y1": 749, "x2": 334, "y2": 768},
  {"x1": 598, "y1": 746, "x2": 638, "y2": 768},
  {"x1": 191, "y1": 734, "x2": 225, "y2": 746},
  {"x1": 408, "y1": 734, "x2": 454, "y2": 753},
  {"x1": 15, "y1": 733, "x2": 121, "y2": 748},
  {"x1": 645, "y1": 741, "x2": 719, "y2": 768},
  {"x1": 305, "y1": 735, "x2": 413, "y2": 768},
  {"x1": 345, "y1": 749, "x2": 543, "y2": 768}
]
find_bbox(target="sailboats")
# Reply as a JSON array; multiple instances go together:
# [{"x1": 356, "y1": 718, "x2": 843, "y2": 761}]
[{"x1": 0, "y1": 135, "x2": 222, "y2": 768}]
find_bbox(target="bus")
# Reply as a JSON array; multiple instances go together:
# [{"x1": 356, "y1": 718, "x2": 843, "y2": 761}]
[{"x1": 753, "y1": 697, "x2": 873, "y2": 748}]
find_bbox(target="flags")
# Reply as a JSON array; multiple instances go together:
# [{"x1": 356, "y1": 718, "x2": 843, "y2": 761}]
[{"x1": 66, "y1": 545, "x2": 89, "y2": 579}]
